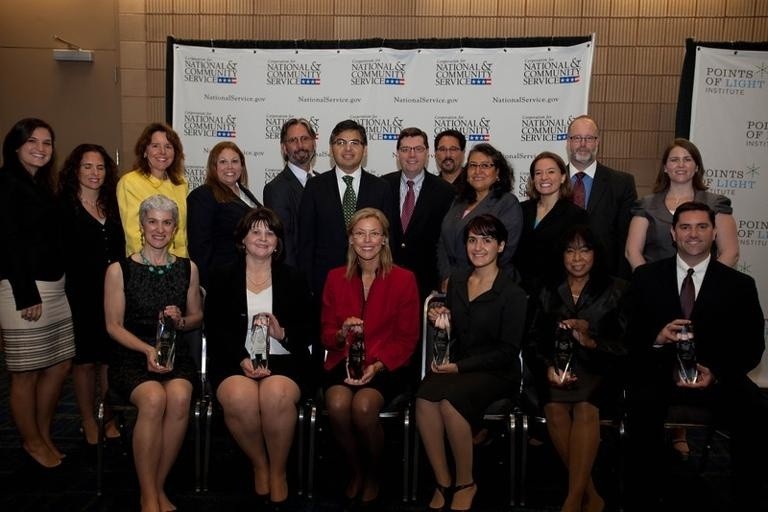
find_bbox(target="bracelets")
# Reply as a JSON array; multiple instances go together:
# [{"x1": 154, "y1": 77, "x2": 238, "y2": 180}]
[{"x1": 179, "y1": 318, "x2": 186, "y2": 329}]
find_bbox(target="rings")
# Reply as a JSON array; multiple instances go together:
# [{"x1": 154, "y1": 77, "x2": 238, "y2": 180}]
[{"x1": 27, "y1": 311, "x2": 32, "y2": 315}]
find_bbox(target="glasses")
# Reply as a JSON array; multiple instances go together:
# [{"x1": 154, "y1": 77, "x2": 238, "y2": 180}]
[
  {"x1": 468, "y1": 162, "x2": 498, "y2": 169},
  {"x1": 438, "y1": 145, "x2": 465, "y2": 154},
  {"x1": 331, "y1": 138, "x2": 365, "y2": 147},
  {"x1": 351, "y1": 230, "x2": 386, "y2": 239},
  {"x1": 398, "y1": 145, "x2": 428, "y2": 153},
  {"x1": 570, "y1": 135, "x2": 597, "y2": 143},
  {"x1": 281, "y1": 136, "x2": 316, "y2": 144}
]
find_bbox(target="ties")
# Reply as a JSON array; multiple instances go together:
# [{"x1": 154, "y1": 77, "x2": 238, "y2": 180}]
[
  {"x1": 680, "y1": 268, "x2": 695, "y2": 319},
  {"x1": 572, "y1": 170, "x2": 586, "y2": 210},
  {"x1": 401, "y1": 181, "x2": 415, "y2": 233},
  {"x1": 342, "y1": 175, "x2": 357, "y2": 231},
  {"x1": 307, "y1": 173, "x2": 312, "y2": 179}
]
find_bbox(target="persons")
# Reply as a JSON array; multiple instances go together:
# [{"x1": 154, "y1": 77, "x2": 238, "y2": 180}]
[
  {"x1": 516, "y1": 151, "x2": 591, "y2": 300},
  {"x1": 103, "y1": 193, "x2": 204, "y2": 511},
  {"x1": 624, "y1": 138, "x2": 740, "y2": 273},
  {"x1": 432, "y1": 129, "x2": 467, "y2": 194},
  {"x1": 114, "y1": 122, "x2": 191, "y2": 263},
  {"x1": 434, "y1": 142, "x2": 524, "y2": 295},
  {"x1": 616, "y1": 201, "x2": 768, "y2": 510},
  {"x1": 300, "y1": 120, "x2": 380, "y2": 279},
  {"x1": 263, "y1": 117, "x2": 321, "y2": 272},
  {"x1": 54, "y1": 142, "x2": 126, "y2": 449},
  {"x1": 318, "y1": 207, "x2": 420, "y2": 501},
  {"x1": 373, "y1": 126, "x2": 451, "y2": 300},
  {"x1": 204, "y1": 207, "x2": 316, "y2": 511},
  {"x1": 183, "y1": 139, "x2": 265, "y2": 435},
  {"x1": 0, "y1": 117, "x2": 79, "y2": 475},
  {"x1": 413, "y1": 212, "x2": 528, "y2": 512},
  {"x1": 523, "y1": 220, "x2": 629, "y2": 511},
  {"x1": 560, "y1": 114, "x2": 638, "y2": 251}
]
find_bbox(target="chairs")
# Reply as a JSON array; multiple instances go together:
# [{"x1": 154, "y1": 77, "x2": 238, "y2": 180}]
[
  {"x1": 412, "y1": 293, "x2": 518, "y2": 511},
  {"x1": 202, "y1": 387, "x2": 306, "y2": 498},
  {"x1": 522, "y1": 413, "x2": 624, "y2": 509},
  {"x1": 308, "y1": 398, "x2": 408, "y2": 505},
  {"x1": 665, "y1": 402, "x2": 711, "y2": 432},
  {"x1": 97, "y1": 285, "x2": 205, "y2": 493}
]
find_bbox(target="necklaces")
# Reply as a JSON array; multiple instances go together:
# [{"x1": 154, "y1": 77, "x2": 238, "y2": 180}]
[
  {"x1": 138, "y1": 248, "x2": 173, "y2": 275},
  {"x1": 246, "y1": 273, "x2": 271, "y2": 286},
  {"x1": 81, "y1": 197, "x2": 100, "y2": 208}
]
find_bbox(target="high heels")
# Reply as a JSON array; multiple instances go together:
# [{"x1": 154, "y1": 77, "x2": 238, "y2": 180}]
[
  {"x1": 449, "y1": 482, "x2": 482, "y2": 512},
  {"x1": 272, "y1": 473, "x2": 290, "y2": 512},
  {"x1": 98, "y1": 419, "x2": 124, "y2": 448},
  {"x1": 342, "y1": 478, "x2": 362, "y2": 505},
  {"x1": 79, "y1": 423, "x2": 106, "y2": 451},
  {"x1": 254, "y1": 470, "x2": 271, "y2": 506},
  {"x1": 670, "y1": 440, "x2": 691, "y2": 466},
  {"x1": 166, "y1": 509, "x2": 178, "y2": 512},
  {"x1": 20, "y1": 444, "x2": 73, "y2": 475},
  {"x1": 357, "y1": 481, "x2": 383, "y2": 508},
  {"x1": 428, "y1": 481, "x2": 451, "y2": 512}
]
